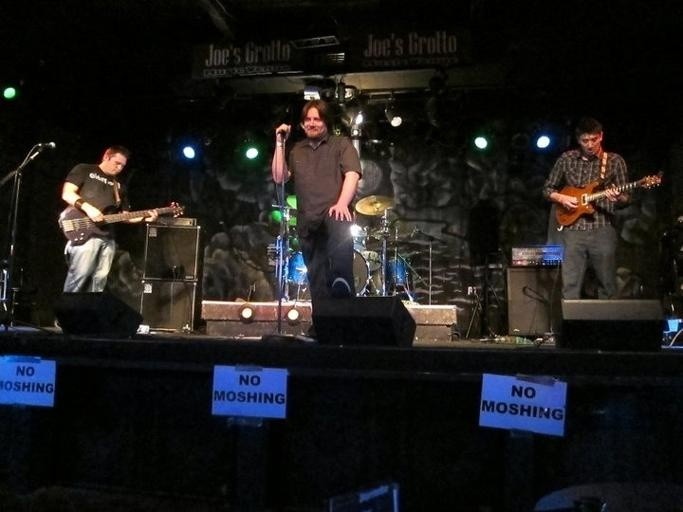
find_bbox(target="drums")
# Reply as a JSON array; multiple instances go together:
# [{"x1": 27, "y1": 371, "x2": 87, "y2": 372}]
[
  {"x1": 351, "y1": 245, "x2": 370, "y2": 298},
  {"x1": 388, "y1": 255, "x2": 415, "y2": 285},
  {"x1": 288, "y1": 252, "x2": 310, "y2": 289}
]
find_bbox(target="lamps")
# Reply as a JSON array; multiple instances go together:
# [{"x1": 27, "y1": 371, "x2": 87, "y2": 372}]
[{"x1": 349, "y1": 66, "x2": 446, "y2": 131}]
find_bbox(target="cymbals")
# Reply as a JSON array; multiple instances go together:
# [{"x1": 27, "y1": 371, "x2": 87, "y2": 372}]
[
  {"x1": 354, "y1": 195, "x2": 397, "y2": 215},
  {"x1": 287, "y1": 194, "x2": 296, "y2": 209}
]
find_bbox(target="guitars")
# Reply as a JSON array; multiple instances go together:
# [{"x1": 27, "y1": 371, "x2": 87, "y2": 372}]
[
  {"x1": 555, "y1": 174, "x2": 660, "y2": 226},
  {"x1": 59, "y1": 201, "x2": 184, "y2": 245}
]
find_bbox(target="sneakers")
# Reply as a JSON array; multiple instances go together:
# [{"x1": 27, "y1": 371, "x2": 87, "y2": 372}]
[{"x1": 328, "y1": 276, "x2": 353, "y2": 300}]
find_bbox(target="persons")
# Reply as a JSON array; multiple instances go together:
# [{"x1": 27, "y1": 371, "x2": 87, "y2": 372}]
[
  {"x1": 541, "y1": 117, "x2": 631, "y2": 298},
  {"x1": 271, "y1": 100, "x2": 362, "y2": 342},
  {"x1": 54, "y1": 144, "x2": 159, "y2": 328}
]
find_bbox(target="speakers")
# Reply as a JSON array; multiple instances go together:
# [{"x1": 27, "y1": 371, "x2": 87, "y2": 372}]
[
  {"x1": 552, "y1": 299, "x2": 664, "y2": 351},
  {"x1": 139, "y1": 281, "x2": 204, "y2": 333},
  {"x1": 143, "y1": 223, "x2": 205, "y2": 281},
  {"x1": 504, "y1": 267, "x2": 562, "y2": 336},
  {"x1": 311, "y1": 298, "x2": 417, "y2": 345},
  {"x1": 52, "y1": 292, "x2": 142, "y2": 337}
]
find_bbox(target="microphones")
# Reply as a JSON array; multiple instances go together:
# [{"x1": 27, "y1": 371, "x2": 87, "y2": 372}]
[{"x1": 38, "y1": 142, "x2": 56, "y2": 149}]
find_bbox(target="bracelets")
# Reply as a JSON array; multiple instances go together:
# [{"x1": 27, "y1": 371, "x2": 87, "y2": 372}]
[
  {"x1": 141, "y1": 216, "x2": 146, "y2": 224},
  {"x1": 74, "y1": 199, "x2": 86, "y2": 208}
]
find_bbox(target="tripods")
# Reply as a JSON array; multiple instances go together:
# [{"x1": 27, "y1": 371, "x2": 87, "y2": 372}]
[
  {"x1": 0, "y1": 148, "x2": 53, "y2": 336},
  {"x1": 366, "y1": 209, "x2": 504, "y2": 341}
]
